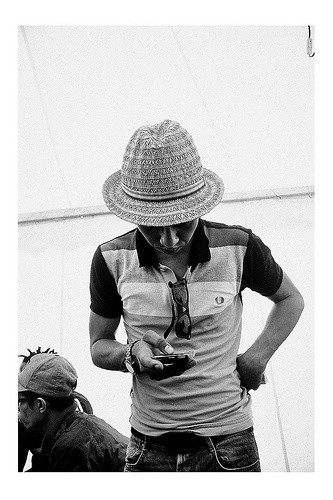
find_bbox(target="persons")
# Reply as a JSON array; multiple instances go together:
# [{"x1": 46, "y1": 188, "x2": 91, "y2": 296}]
[
  {"x1": 18, "y1": 352, "x2": 129, "y2": 472},
  {"x1": 86, "y1": 119, "x2": 305, "y2": 472}
]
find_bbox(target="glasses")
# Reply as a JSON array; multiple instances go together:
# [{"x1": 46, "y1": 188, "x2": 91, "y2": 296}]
[{"x1": 169, "y1": 277, "x2": 192, "y2": 339}]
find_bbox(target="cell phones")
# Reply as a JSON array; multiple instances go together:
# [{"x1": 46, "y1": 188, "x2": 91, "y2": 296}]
[{"x1": 152, "y1": 352, "x2": 189, "y2": 363}]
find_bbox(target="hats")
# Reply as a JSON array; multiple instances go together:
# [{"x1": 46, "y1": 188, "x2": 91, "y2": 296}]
[
  {"x1": 101, "y1": 120, "x2": 224, "y2": 226},
  {"x1": 18, "y1": 354, "x2": 93, "y2": 414}
]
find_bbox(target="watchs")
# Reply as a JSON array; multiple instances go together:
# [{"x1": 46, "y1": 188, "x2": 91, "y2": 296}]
[{"x1": 124, "y1": 339, "x2": 141, "y2": 376}]
